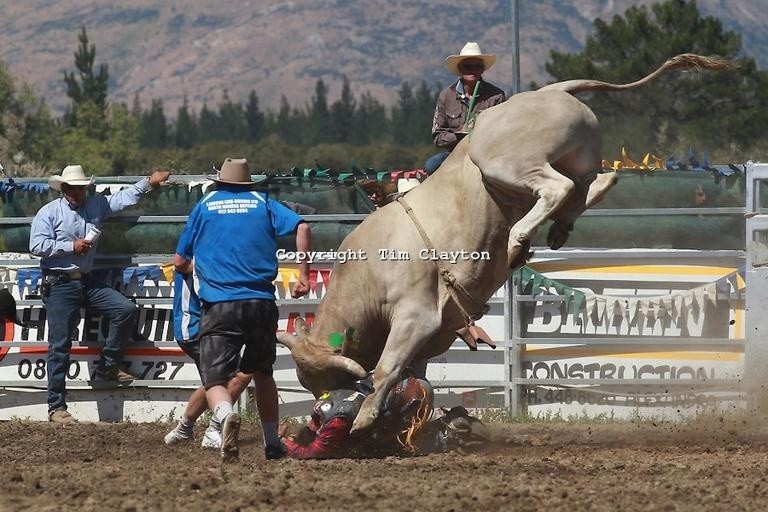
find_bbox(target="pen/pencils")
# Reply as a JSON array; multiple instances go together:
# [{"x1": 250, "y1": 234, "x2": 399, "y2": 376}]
[{"x1": 81, "y1": 236, "x2": 91, "y2": 247}]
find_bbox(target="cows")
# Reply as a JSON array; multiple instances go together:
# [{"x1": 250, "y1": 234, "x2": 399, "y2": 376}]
[{"x1": 272, "y1": 53, "x2": 748, "y2": 443}]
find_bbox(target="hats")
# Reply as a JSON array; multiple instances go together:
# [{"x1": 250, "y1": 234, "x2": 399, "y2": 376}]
[
  {"x1": 348, "y1": 178, "x2": 420, "y2": 210},
  {"x1": 204, "y1": 157, "x2": 266, "y2": 184},
  {"x1": 444, "y1": 41, "x2": 496, "y2": 72},
  {"x1": 48, "y1": 164, "x2": 96, "y2": 191}
]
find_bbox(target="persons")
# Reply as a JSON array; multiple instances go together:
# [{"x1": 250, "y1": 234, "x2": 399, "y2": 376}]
[
  {"x1": 424, "y1": 42, "x2": 507, "y2": 177},
  {"x1": 689, "y1": 181, "x2": 718, "y2": 207},
  {"x1": 171, "y1": 158, "x2": 315, "y2": 461},
  {"x1": 161, "y1": 255, "x2": 257, "y2": 451},
  {"x1": 27, "y1": 164, "x2": 172, "y2": 426},
  {"x1": 279, "y1": 367, "x2": 493, "y2": 462},
  {"x1": 357, "y1": 178, "x2": 396, "y2": 208}
]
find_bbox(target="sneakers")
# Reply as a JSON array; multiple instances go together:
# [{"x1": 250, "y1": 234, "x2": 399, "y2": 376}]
[
  {"x1": 165, "y1": 431, "x2": 188, "y2": 445},
  {"x1": 50, "y1": 410, "x2": 75, "y2": 423},
  {"x1": 220, "y1": 412, "x2": 241, "y2": 463},
  {"x1": 448, "y1": 406, "x2": 490, "y2": 441},
  {"x1": 201, "y1": 433, "x2": 222, "y2": 449},
  {"x1": 265, "y1": 443, "x2": 287, "y2": 460},
  {"x1": 103, "y1": 367, "x2": 139, "y2": 382}
]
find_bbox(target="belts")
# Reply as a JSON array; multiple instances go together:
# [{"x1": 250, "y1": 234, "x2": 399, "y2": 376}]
[{"x1": 43, "y1": 271, "x2": 83, "y2": 280}]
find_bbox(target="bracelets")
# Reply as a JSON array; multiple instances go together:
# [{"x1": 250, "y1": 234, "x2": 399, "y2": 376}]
[{"x1": 145, "y1": 176, "x2": 151, "y2": 184}]
